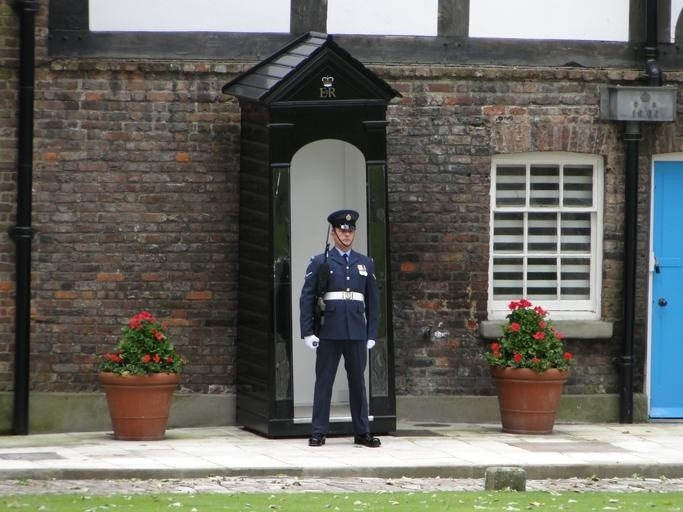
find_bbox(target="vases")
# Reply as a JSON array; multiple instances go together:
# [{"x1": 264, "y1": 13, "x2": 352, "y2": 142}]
[
  {"x1": 98, "y1": 372, "x2": 179, "y2": 441},
  {"x1": 489, "y1": 366, "x2": 569, "y2": 434}
]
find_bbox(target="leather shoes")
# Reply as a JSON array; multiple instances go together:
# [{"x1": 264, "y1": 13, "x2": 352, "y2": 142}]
[
  {"x1": 354, "y1": 433, "x2": 381, "y2": 447},
  {"x1": 309, "y1": 433, "x2": 325, "y2": 446}
]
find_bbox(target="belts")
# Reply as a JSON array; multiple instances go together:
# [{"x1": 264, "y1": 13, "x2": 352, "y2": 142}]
[{"x1": 322, "y1": 291, "x2": 365, "y2": 300}]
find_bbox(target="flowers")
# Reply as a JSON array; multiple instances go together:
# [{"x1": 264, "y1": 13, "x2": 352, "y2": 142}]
[
  {"x1": 483, "y1": 299, "x2": 574, "y2": 373},
  {"x1": 94, "y1": 312, "x2": 190, "y2": 378}
]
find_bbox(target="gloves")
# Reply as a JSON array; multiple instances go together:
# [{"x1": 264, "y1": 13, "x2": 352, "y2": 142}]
[
  {"x1": 366, "y1": 338, "x2": 375, "y2": 349},
  {"x1": 303, "y1": 335, "x2": 320, "y2": 349}
]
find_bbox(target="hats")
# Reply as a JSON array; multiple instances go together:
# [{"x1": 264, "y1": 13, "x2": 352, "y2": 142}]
[{"x1": 327, "y1": 210, "x2": 359, "y2": 227}]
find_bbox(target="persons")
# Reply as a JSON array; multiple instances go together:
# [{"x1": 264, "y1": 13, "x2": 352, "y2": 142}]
[{"x1": 298, "y1": 210, "x2": 383, "y2": 447}]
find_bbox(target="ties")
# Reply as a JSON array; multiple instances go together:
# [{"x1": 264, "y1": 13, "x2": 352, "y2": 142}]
[{"x1": 343, "y1": 254, "x2": 349, "y2": 264}]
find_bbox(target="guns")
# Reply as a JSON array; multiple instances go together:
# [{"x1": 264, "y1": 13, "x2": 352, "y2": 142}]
[{"x1": 312, "y1": 224, "x2": 330, "y2": 345}]
